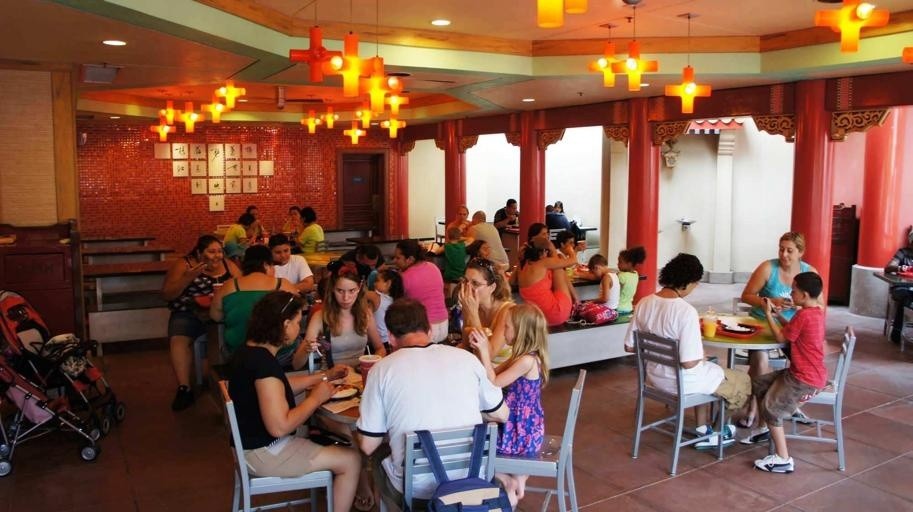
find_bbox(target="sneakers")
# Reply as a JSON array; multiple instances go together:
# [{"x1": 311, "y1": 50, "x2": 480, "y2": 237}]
[
  {"x1": 740, "y1": 427, "x2": 770, "y2": 445},
  {"x1": 891, "y1": 330, "x2": 901, "y2": 344},
  {"x1": 172, "y1": 384, "x2": 194, "y2": 411},
  {"x1": 695, "y1": 425, "x2": 736, "y2": 450},
  {"x1": 724, "y1": 424, "x2": 736, "y2": 439},
  {"x1": 755, "y1": 454, "x2": 794, "y2": 474}
]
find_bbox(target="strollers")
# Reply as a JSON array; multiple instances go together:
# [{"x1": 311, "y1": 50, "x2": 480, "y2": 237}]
[{"x1": 0, "y1": 290, "x2": 126, "y2": 477}]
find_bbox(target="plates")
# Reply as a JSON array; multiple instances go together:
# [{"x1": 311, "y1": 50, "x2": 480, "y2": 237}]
[
  {"x1": 700, "y1": 320, "x2": 766, "y2": 339},
  {"x1": 326, "y1": 385, "x2": 358, "y2": 403}
]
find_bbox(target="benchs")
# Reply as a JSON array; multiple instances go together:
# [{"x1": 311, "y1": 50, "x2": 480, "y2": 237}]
[
  {"x1": 89, "y1": 303, "x2": 178, "y2": 354},
  {"x1": 537, "y1": 312, "x2": 634, "y2": 369}
]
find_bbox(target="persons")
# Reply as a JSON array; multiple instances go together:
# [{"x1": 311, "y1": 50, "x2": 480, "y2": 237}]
[
  {"x1": 455, "y1": 256, "x2": 516, "y2": 370},
  {"x1": 575, "y1": 254, "x2": 621, "y2": 312},
  {"x1": 246, "y1": 205, "x2": 265, "y2": 239},
  {"x1": 468, "y1": 304, "x2": 549, "y2": 507},
  {"x1": 556, "y1": 230, "x2": 575, "y2": 261},
  {"x1": 545, "y1": 205, "x2": 571, "y2": 249},
  {"x1": 362, "y1": 269, "x2": 404, "y2": 357},
  {"x1": 288, "y1": 207, "x2": 324, "y2": 254},
  {"x1": 623, "y1": 252, "x2": 737, "y2": 450},
  {"x1": 518, "y1": 222, "x2": 566, "y2": 270},
  {"x1": 465, "y1": 239, "x2": 490, "y2": 258},
  {"x1": 739, "y1": 271, "x2": 830, "y2": 473},
  {"x1": 305, "y1": 267, "x2": 387, "y2": 371},
  {"x1": 221, "y1": 290, "x2": 361, "y2": 512},
  {"x1": 327, "y1": 244, "x2": 388, "y2": 285},
  {"x1": 354, "y1": 298, "x2": 509, "y2": 512},
  {"x1": 494, "y1": 199, "x2": 520, "y2": 240},
  {"x1": 883, "y1": 226, "x2": 913, "y2": 343},
  {"x1": 737, "y1": 231, "x2": 825, "y2": 429},
  {"x1": 269, "y1": 234, "x2": 314, "y2": 293},
  {"x1": 616, "y1": 246, "x2": 646, "y2": 316},
  {"x1": 518, "y1": 241, "x2": 582, "y2": 326},
  {"x1": 466, "y1": 211, "x2": 510, "y2": 272},
  {"x1": 554, "y1": 201, "x2": 564, "y2": 213},
  {"x1": 280, "y1": 206, "x2": 303, "y2": 233},
  {"x1": 393, "y1": 239, "x2": 448, "y2": 344},
  {"x1": 447, "y1": 206, "x2": 471, "y2": 241},
  {"x1": 436, "y1": 227, "x2": 474, "y2": 298},
  {"x1": 207, "y1": 245, "x2": 300, "y2": 415},
  {"x1": 160, "y1": 234, "x2": 242, "y2": 412},
  {"x1": 222, "y1": 213, "x2": 257, "y2": 262}
]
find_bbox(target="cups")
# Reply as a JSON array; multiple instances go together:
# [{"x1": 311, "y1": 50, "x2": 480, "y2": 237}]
[
  {"x1": 358, "y1": 355, "x2": 381, "y2": 390},
  {"x1": 701, "y1": 311, "x2": 719, "y2": 338}
]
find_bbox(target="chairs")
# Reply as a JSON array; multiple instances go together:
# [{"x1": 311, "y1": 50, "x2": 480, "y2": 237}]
[
  {"x1": 727, "y1": 294, "x2": 790, "y2": 377},
  {"x1": 548, "y1": 227, "x2": 564, "y2": 252},
  {"x1": 432, "y1": 214, "x2": 448, "y2": 243},
  {"x1": 211, "y1": 380, "x2": 336, "y2": 512},
  {"x1": 401, "y1": 426, "x2": 503, "y2": 512},
  {"x1": 499, "y1": 367, "x2": 589, "y2": 512},
  {"x1": 630, "y1": 327, "x2": 727, "y2": 478},
  {"x1": 774, "y1": 326, "x2": 854, "y2": 468},
  {"x1": 303, "y1": 348, "x2": 327, "y2": 372}
]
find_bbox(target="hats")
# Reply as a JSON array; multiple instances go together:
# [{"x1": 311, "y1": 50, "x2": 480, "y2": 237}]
[{"x1": 530, "y1": 238, "x2": 552, "y2": 249}]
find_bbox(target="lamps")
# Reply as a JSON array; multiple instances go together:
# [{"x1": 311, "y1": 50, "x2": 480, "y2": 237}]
[
  {"x1": 214, "y1": 80, "x2": 246, "y2": 108},
  {"x1": 610, "y1": 5, "x2": 662, "y2": 91},
  {"x1": 159, "y1": 101, "x2": 180, "y2": 124},
  {"x1": 200, "y1": 94, "x2": 232, "y2": 125},
  {"x1": 385, "y1": 93, "x2": 410, "y2": 115},
  {"x1": 588, "y1": 25, "x2": 623, "y2": 87},
  {"x1": 379, "y1": 114, "x2": 406, "y2": 139},
  {"x1": 354, "y1": 102, "x2": 378, "y2": 129},
  {"x1": 359, "y1": 0, "x2": 398, "y2": 114},
  {"x1": 660, "y1": 16, "x2": 709, "y2": 115},
  {"x1": 288, "y1": 0, "x2": 342, "y2": 83},
  {"x1": 177, "y1": 102, "x2": 205, "y2": 134},
  {"x1": 534, "y1": 0, "x2": 565, "y2": 29},
  {"x1": 149, "y1": 117, "x2": 178, "y2": 143},
  {"x1": 299, "y1": 110, "x2": 322, "y2": 135},
  {"x1": 319, "y1": 107, "x2": 340, "y2": 129},
  {"x1": 560, "y1": 0, "x2": 587, "y2": 15},
  {"x1": 809, "y1": 0, "x2": 890, "y2": 55},
  {"x1": 902, "y1": 46, "x2": 913, "y2": 64},
  {"x1": 343, "y1": 122, "x2": 366, "y2": 145},
  {"x1": 322, "y1": 0, "x2": 382, "y2": 99}
]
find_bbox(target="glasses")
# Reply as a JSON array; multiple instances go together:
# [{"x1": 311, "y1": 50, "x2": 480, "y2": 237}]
[
  {"x1": 282, "y1": 289, "x2": 304, "y2": 312},
  {"x1": 470, "y1": 258, "x2": 496, "y2": 282}
]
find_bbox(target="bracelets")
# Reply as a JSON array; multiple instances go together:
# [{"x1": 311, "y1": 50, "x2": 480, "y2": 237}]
[
  {"x1": 897, "y1": 266, "x2": 901, "y2": 272},
  {"x1": 320, "y1": 372, "x2": 328, "y2": 381}
]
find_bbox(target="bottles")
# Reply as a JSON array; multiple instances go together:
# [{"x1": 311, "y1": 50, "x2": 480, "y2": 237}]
[{"x1": 255, "y1": 232, "x2": 268, "y2": 248}]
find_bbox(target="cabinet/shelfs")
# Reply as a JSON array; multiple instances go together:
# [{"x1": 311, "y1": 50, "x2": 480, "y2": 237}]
[
  {"x1": 81, "y1": 247, "x2": 173, "y2": 260},
  {"x1": 81, "y1": 235, "x2": 154, "y2": 245},
  {"x1": 80, "y1": 257, "x2": 176, "y2": 297}
]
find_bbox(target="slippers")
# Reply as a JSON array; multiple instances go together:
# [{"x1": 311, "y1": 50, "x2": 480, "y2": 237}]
[
  {"x1": 737, "y1": 415, "x2": 756, "y2": 429},
  {"x1": 792, "y1": 413, "x2": 813, "y2": 425},
  {"x1": 355, "y1": 494, "x2": 373, "y2": 511}
]
